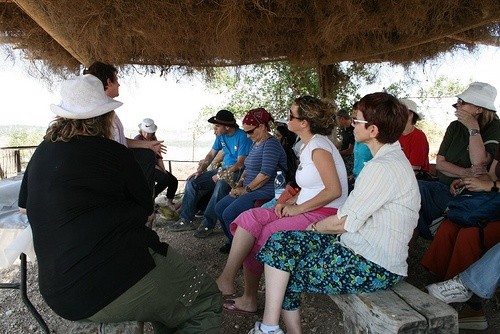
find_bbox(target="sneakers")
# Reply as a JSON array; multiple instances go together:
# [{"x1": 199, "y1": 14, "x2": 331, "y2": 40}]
[
  {"x1": 425, "y1": 275, "x2": 473, "y2": 304},
  {"x1": 456, "y1": 311, "x2": 488, "y2": 330},
  {"x1": 193, "y1": 225, "x2": 213, "y2": 237},
  {"x1": 167, "y1": 219, "x2": 195, "y2": 231}
]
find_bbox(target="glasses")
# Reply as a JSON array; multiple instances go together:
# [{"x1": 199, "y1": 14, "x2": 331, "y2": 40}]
[
  {"x1": 289, "y1": 110, "x2": 302, "y2": 121},
  {"x1": 458, "y1": 98, "x2": 470, "y2": 104},
  {"x1": 245, "y1": 126, "x2": 258, "y2": 134},
  {"x1": 353, "y1": 118, "x2": 368, "y2": 124}
]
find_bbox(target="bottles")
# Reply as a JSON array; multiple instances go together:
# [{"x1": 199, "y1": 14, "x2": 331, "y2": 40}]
[{"x1": 273, "y1": 172, "x2": 287, "y2": 200}]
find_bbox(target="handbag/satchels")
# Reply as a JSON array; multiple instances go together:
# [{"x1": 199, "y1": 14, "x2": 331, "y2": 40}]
[{"x1": 443, "y1": 188, "x2": 500, "y2": 227}]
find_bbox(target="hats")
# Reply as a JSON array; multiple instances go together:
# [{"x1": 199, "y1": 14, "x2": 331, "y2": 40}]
[
  {"x1": 455, "y1": 81, "x2": 497, "y2": 111},
  {"x1": 50, "y1": 74, "x2": 123, "y2": 119},
  {"x1": 400, "y1": 98, "x2": 422, "y2": 121},
  {"x1": 336, "y1": 109, "x2": 351, "y2": 119},
  {"x1": 138, "y1": 118, "x2": 158, "y2": 133},
  {"x1": 208, "y1": 110, "x2": 239, "y2": 128}
]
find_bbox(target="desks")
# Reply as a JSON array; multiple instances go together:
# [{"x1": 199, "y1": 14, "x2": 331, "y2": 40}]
[{"x1": 0, "y1": 171, "x2": 52, "y2": 334}]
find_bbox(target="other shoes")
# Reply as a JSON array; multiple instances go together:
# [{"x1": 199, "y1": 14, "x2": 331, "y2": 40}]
[
  {"x1": 247, "y1": 321, "x2": 284, "y2": 334},
  {"x1": 156, "y1": 206, "x2": 176, "y2": 217},
  {"x1": 159, "y1": 215, "x2": 179, "y2": 222},
  {"x1": 220, "y1": 243, "x2": 231, "y2": 253}
]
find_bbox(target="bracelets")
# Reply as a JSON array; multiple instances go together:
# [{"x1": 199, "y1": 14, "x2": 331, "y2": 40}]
[
  {"x1": 217, "y1": 173, "x2": 220, "y2": 179},
  {"x1": 490, "y1": 181, "x2": 499, "y2": 193}
]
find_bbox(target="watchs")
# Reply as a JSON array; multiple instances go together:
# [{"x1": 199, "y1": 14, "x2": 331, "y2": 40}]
[
  {"x1": 246, "y1": 185, "x2": 253, "y2": 192},
  {"x1": 310, "y1": 221, "x2": 318, "y2": 231},
  {"x1": 468, "y1": 129, "x2": 480, "y2": 136}
]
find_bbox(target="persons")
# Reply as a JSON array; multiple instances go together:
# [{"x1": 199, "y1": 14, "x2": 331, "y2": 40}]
[
  {"x1": 215, "y1": 108, "x2": 288, "y2": 254},
  {"x1": 167, "y1": 110, "x2": 253, "y2": 238},
  {"x1": 414, "y1": 82, "x2": 500, "y2": 330},
  {"x1": 398, "y1": 98, "x2": 429, "y2": 173},
  {"x1": 215, "y1": 95, "x2": 348, "y2": 314},
  {"x1": 335, "y1": 108, "x2": 355, "y2": 169},
  {"x1": 88, "y1": 61, "x2": 167, "y2": 158},
  {"x1": 133, "y1": 118, "x2": 178, "y2": 229},
  {"x1": 348, "y1": 101, "x2": 374, "y2": 196},
  {"x1": 248, "y1": 92, "x2": 422, "y2": 334},
  {"x1": 17, "y1": 74, "x2": 224, "y2": 334}
]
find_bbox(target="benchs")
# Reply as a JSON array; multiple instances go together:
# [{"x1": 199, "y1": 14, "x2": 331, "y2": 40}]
[{"x1": 324, "y1": 281, "x2": 457, "y2": 334}]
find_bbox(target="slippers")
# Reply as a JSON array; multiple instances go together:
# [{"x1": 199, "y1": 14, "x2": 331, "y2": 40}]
[{"x1": 222, "y1": 299, "x2": 259, "y2": 314}]
